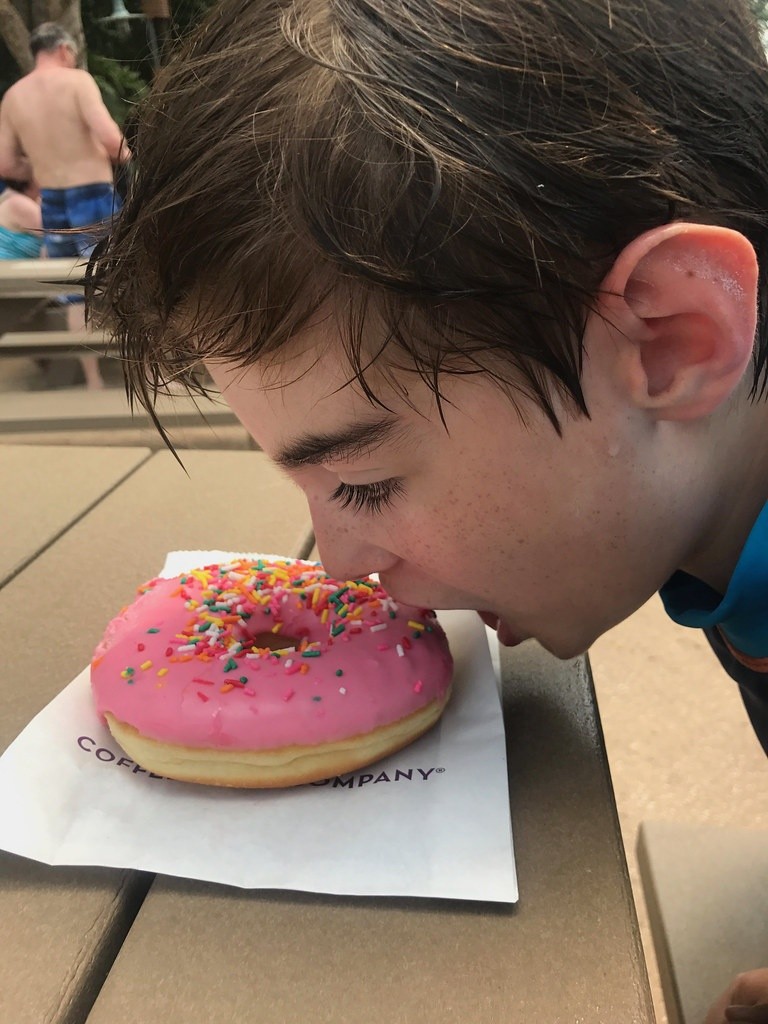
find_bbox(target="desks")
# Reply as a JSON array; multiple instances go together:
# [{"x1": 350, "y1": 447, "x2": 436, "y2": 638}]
[
  {"x1": 0, "y1": 441, "x2": 661, "y2": 1024},
  {"x1": 0, "y1": 259, "x2": 101, "y2": 301}
]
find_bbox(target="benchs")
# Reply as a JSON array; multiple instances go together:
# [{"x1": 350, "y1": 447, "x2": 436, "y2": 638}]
[
  {"x1": 0, "y1": 329, "x2": 137, "y2": 361},
  {"x1": 0, "y1": 383, "x2": 260, "y2": 452},
  {"x1": 636, "y1": 817, "x2": 768, "y2": 1024}
]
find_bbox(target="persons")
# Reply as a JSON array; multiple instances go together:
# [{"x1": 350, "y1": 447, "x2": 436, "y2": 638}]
[
  {"x1": 0, "y1": 20, "x2": 185, "y2": 394},
  {"x1": 24, "y1": 0, "x2": 767, "y2": 1024}
]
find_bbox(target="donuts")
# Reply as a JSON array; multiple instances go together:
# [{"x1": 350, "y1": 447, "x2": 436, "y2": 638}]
[{"x1": 90, "y1": 558, "x2": 458, "y2": 790}]
